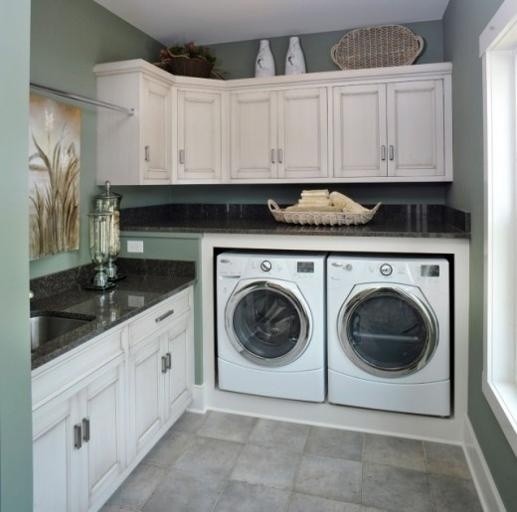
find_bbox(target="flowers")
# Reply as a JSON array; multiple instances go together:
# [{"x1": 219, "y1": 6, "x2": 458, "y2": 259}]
[{"x1": 158, "y1": 42, "x2": 219, "y2": 61}]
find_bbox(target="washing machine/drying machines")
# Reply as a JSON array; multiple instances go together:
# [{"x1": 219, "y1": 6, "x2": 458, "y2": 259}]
[
  {"x1": 216, "y1": 253, "x2": 327, "y2": 404},
  {"x1": 326, "y1": 255, "x2": 451, "y2": 418}
]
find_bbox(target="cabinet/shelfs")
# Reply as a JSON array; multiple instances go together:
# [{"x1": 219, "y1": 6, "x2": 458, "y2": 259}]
[
  {"x1": 31, "y1": 328, "x2": 134, "y2": 512},
  {"x1": 121, "y1": 286, "x2": 195, "y2": 461},
  {"x1": 94, "y1": 59, "x2": 453, "y2": 186}
]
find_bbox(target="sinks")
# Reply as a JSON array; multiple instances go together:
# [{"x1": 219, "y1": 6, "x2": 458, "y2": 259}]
[{"x1": 31, "y1": 310, "x2": 96, "y2": 351}]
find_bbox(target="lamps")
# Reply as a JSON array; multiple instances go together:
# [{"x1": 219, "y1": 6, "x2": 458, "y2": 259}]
[
  {"x1": 94, "y1": 181, "x2": 127, "y2": 282},
  {"x1": 86, "y1": 198, "x2": 116, "y2": 290}
]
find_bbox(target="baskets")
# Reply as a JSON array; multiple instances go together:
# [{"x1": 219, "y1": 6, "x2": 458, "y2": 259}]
[
  {"x1": 329, "y1": 23, "x2": 426, "y2": 71},
  {"x1": 152, "y1": 58, "x2": 216, "y2": 78},
  {"x1": 266, "y1": 197, "x2": 384, "y2": 226}
]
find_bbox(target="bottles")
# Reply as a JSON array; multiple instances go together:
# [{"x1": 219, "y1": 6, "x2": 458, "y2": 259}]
[
  {"x1": 255, "y1": 36, "x2": 306, "y2": 77},
  {"x1": 84, "y1": 182, "x2": 126, "y2": 292}
]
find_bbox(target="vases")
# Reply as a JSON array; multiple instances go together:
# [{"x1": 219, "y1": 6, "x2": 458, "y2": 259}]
[
  {"x1": 285, "y1": 37, "x2": 306, "y2": 75},
  {"x1": 255, "y1": 40, "x2": 275, "y2": 77}
]
